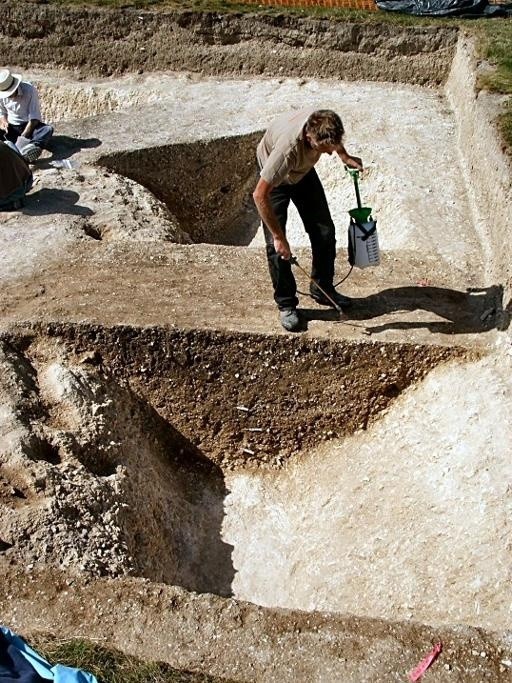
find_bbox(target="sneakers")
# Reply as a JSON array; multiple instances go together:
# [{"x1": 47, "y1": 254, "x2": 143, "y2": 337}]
[
  {"x1": 310, "y1": 292, "x2": 352, "y2": 307},
  {"x1": 279, "y1": 306, "x2": 299, "y2": 330},
  {"x1": 20, "y1": 146, "x2": 41, "y2": 162}
]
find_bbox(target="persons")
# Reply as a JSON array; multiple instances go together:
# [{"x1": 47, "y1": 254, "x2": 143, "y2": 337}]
[
  {"x1": 0, "y1": 67, "x2": 55, "y2": 161},
  {"x1": 247, "y1": 102, "x2": 366, "y2": 329},
  {"x1": 0, "y1": 138, "x2": 35, "y2": 212}
]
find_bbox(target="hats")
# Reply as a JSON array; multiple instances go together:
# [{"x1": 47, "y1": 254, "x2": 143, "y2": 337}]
[{"x1": 0, "y1": 69, "x2": 22, "y2": 98}]
